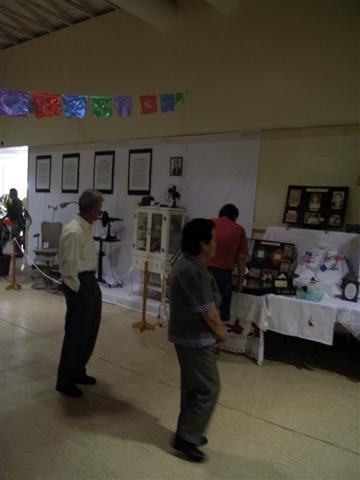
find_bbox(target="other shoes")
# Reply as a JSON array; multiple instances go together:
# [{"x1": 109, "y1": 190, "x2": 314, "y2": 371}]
[
  {"x1": 75, "y1": 377, "x2": 97, "y2": 384},
  {"x1": 56, "y1": 383, "x2": 82, "y2": 398},
  {"x1": 199, "y1": 436, "x2": 207, "y2": 445},
  {"x1": 173, "y1": 438, "x2": 204, "y2": 461}
]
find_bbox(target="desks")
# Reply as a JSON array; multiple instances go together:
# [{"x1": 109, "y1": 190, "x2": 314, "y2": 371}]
[{"x1": 216, "y1": 223, "x2": 360, "y2": 381}]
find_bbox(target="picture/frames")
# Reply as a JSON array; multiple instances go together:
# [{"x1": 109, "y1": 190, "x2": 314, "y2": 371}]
[{"x1": 169, "y1": 157, "x2": 183, "y2": 176}]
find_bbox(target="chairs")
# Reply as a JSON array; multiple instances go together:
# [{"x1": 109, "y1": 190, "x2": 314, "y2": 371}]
[{"x1": 31, "y1": 221, "x2": 63, "y2": 277}]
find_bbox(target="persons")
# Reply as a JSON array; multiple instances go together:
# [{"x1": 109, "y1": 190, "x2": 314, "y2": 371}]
[
  {"x1": 3, "y1": 188, "x2": 23, "y2": 257},
  {"x1": 332, "y1": 193, "x2": 342, "y2": 210},
  {"x1": 167, "y1": 218, "x2": 228, "y2": 461},
  {"x1": 57, "y1": 190, "x2": 104, "y2": 398},
  {"x1": 208, "y1": 203, "x2": 247, "y2": 324}
]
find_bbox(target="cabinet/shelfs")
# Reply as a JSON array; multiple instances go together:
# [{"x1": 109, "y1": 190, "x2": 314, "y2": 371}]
[
  {"x1": 281, "y1": 184, "x2": 350, "y2": 230},
  {"x1": 127, "y1": 205, "x2": 188, "y2": 298}
]
